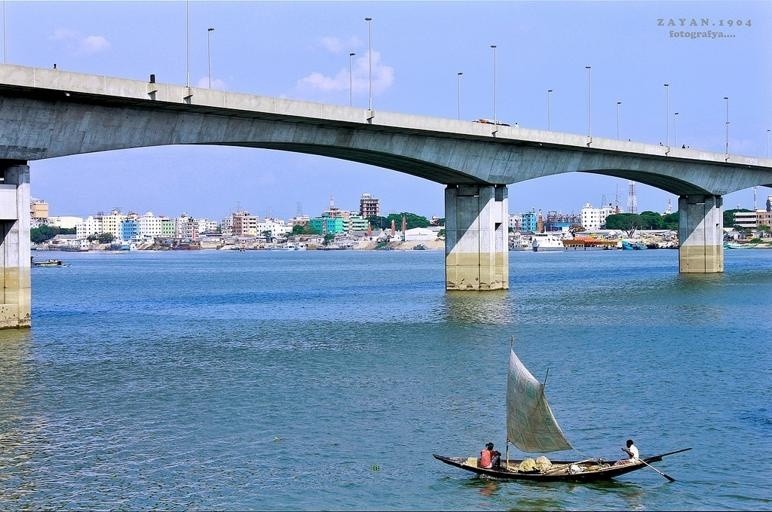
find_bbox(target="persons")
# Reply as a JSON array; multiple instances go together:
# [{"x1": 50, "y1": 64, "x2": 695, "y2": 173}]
[
  {"x1": 613, "y1": 439, "x2": 639, "y2": 466},
  {"x1": 485, "y1": 443, "x2": 489, "y2": 449},
  {"x1": 479, "y1": 442, "x2": 501, "y2": 470}
]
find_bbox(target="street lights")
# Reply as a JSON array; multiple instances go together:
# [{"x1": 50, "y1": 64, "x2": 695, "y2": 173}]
[
  {"x1": 768, "y1": 130, "x2": 770, "y2": 158},
  {"x1": 208, "y1": 28, "x2": 214, "y2": 89},
  {"x1": 617, "y1": 102, "x2": 621, "y2": 141},
  {"x1": 675, "y1": 113, "x2": 678, "y2": 146},
  {"x1": 724, "y1": 97, "x2": 731, "y2": 153},
  {"x1": 664, "y1": 84, "x2": 670, "y2": 147},
  {"x1": 586, "y1": 67, "x2": 591, "y2": 136},
  {"x1": 491, "y1": 46, "x2": 496, "y2": 126},
  {"x1": 350, "y1": 53, "x2": 355, "y2": 107},
  {"x1": 365, "y1": 18, "x2": 372, "y2": 108},
  {"x1": 458, "y1": 73, "x2": 462, "y2": 120},
  {"x1": 548, "y1": 90, "x2": 553, "y2": 132}
]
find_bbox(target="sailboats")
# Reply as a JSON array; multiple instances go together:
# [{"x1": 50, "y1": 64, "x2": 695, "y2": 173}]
[{"x1": 433, "y1": 335, "x2": 661, "y2": 482}]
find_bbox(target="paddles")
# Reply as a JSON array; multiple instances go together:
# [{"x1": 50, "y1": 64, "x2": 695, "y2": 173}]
[{"x1": 620, "y1": 447, "x2": 675, "y2": 482}]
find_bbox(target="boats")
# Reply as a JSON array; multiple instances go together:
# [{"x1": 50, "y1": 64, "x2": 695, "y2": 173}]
[
  {"x1": 34, "y1": 260, "x2": 62, "y2": 267},
  {"x1": 34, "y1": 258, "x2": 63, "y2": 267}
]
